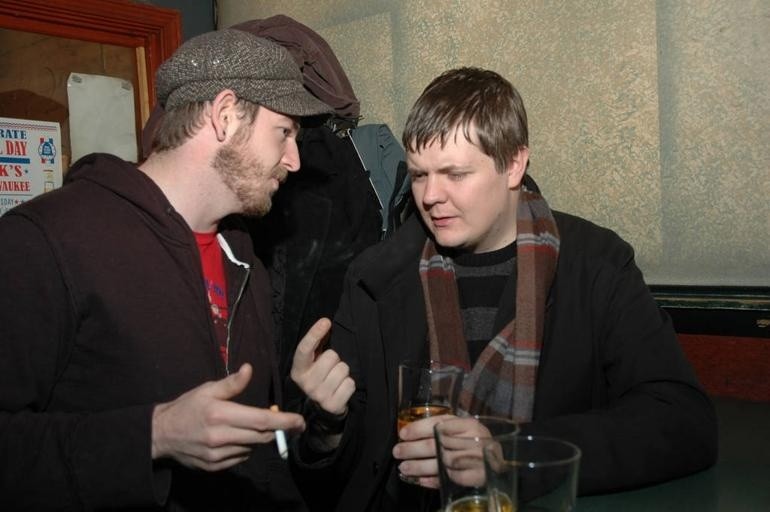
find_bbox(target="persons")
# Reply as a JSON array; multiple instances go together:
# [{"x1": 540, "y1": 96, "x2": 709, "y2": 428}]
[
  {"x1": 286, "y1": 65, "x2": 718, "y2": 511},
  {"x1": 1, "y1": 28, "x2": 333, "y2": 511}
]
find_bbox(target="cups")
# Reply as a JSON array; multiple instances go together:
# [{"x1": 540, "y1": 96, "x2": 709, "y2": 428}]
[
  {"x1": 396, "y1": 360, "x2": 465, "y2": 487},
  {"x1": 433, "y1": 415, "x2": 523, "y2": 512},
  {"x1": 483, "y1": 435, "x2": 582, "y2": 511}
]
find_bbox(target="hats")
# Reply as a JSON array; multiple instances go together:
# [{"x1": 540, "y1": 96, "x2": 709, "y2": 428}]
[{"x1": 155, "y1": 29, "x2": 337, "y2": 121}]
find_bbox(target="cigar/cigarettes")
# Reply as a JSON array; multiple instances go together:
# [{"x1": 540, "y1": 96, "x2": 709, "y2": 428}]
[{"x1": 269, "y1": 405, "x2": 289, "y2": 460}]
